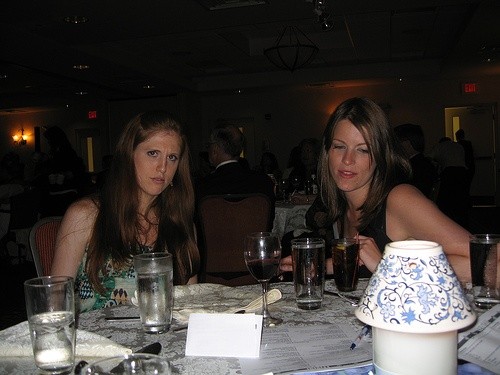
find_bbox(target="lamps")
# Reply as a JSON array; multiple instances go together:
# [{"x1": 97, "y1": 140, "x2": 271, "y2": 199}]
[
  {"x1": 310, "y1": 0, "x2": 337, "y2": 33},
  {"x1": 12, "y1": 128, "x2": 31, "y2": 145},
  {"x1": 264, "y1": 24, "x2": 318, "y2": 73}
]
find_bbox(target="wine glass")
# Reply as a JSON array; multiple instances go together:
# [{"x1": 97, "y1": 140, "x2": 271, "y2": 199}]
[{"x1": 242, "y1": 230, "x2": 283, "y2": 327}]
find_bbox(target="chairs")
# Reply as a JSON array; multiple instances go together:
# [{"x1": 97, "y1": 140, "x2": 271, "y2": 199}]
[
  {"x1": 28, "y1": 215, "x2": 63, "y2": 275},
  {"x1": 197, "y1": 192, "x2": 271, "y2": 283}
]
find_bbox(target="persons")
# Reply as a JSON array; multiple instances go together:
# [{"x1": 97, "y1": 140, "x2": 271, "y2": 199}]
[
  {"x1": 0, "y1": 123, "x2": 475, "y2": 281},
  {"x1": 274, "y1": 97, "x2": 478, "y2": 283},
  {"x1": 50, "y1": 110, "x2": 200, "y2": 313}
]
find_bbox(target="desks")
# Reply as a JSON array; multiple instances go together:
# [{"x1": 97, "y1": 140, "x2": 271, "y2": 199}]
[
  {"x1": 0, "y1": 272, "x2": 500, "y2": 375},
  {"x1": 271, "y1": 196, "x2": 315, "y2": 237}
]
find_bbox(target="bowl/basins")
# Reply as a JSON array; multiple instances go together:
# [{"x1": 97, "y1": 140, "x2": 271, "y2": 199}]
[
  {"x1": 85, "y1": 351, "x2": 174, "y2": 375},
  {"x1": 354, "y1": 240, "x2": 477, "y2": 333}
]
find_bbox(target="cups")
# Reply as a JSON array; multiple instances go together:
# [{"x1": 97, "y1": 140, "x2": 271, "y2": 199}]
[
  {"x1": 290, "y1": 238, "x2": 325, "y2": 310},
  {"x1": 469, "y1": 233, "x2": 500, "y2": 311},
  {"x1": 23, "y1": 275, "x2": 77, "y2": 375},
  {"x1": 280, "y1": 173, "x2": 319, "y2": 205},
  {"x1": 332, "y1": 234, "x2": 361, "y2": 292},
  {"x1": 134, "y1": 252, "x2": 175, "y2": 333}
]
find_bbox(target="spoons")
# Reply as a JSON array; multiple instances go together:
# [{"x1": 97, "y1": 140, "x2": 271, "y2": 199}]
[{"x1": 74, "y1": 342, "x2": 162, "y2": 375}]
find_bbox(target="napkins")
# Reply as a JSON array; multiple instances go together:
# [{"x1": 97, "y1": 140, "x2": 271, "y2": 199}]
[
  {"x1": 132, "y1": 283, "x2": 282, "y2": 323},
  {"x1": 0, "y1": 320, "x2": 132, "y2": 358}
]
